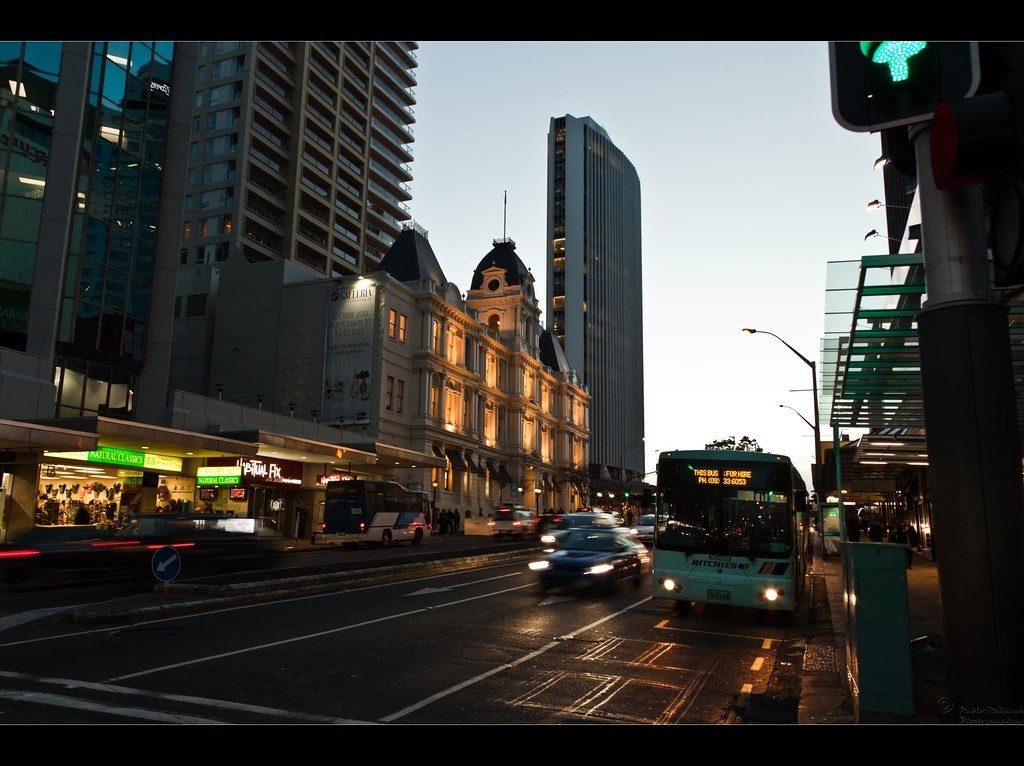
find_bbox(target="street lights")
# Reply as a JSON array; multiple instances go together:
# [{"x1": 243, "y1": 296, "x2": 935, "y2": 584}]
[
  {"x1": 742, "y1": 326, "x2": 822, "y2": 491},
  {"x1": 778, "y1": 405, "x2": 817, "y2": 471}
]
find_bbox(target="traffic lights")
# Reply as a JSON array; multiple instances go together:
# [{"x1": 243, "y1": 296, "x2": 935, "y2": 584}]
[{"x1": 656, "y1": 463, "x2": 658, "y2": 475}]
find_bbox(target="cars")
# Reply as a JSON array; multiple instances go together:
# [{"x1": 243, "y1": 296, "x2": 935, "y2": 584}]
[
  {"x1": 535, "y1": 506, "x2": 625, "y2": 539},
  {"x1": 538, "y1": 527, "x2": 651, "y2": 601},
  {"x1": 630, "y1": 513, "x2": 667, "y2": 545},
  {"x1": 490, "y1": 510, "x2": 540, "y2": 540}
]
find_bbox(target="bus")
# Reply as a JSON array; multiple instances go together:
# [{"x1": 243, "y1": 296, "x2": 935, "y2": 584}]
[
  {"x1": 650, "y1": 450, "x2": 820, "y2": 619},
  {"x1": 319, "y1": 479, "x2": 432, "y2": 550}
]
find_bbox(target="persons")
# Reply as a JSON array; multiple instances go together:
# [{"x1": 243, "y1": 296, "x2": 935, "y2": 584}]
[
  {"x1": 74, "y1": 503, "x2": 90, "y2": 525},
  {"x1": 541, "y1": 506, "x2": 564, "y2": 514},
  {"x1": 439, "y1": 508, "x2": 460, "y2": 535},
  {"x1": 628, "y1": 510, "x2": 633, "y2": 525},
  {"x1": 847, "y1": 516, "x2": 919, "y2": 571}
]
